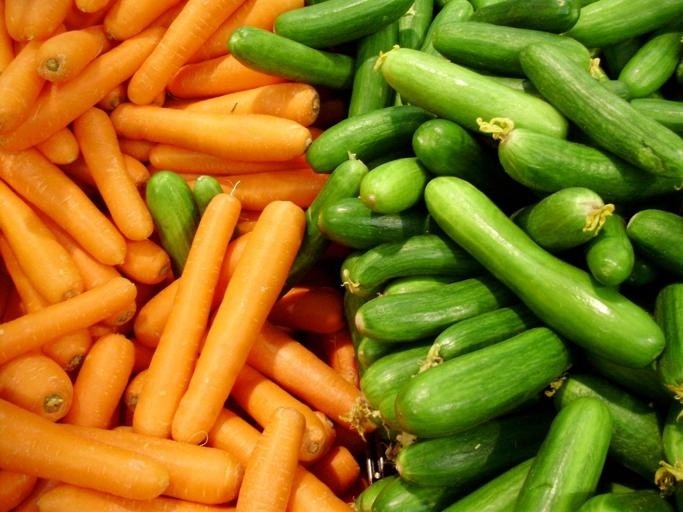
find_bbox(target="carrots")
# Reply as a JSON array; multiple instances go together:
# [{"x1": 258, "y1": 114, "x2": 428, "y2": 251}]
[{"x1": 0, "y1": 0, "x2": 378, "y2": 512}]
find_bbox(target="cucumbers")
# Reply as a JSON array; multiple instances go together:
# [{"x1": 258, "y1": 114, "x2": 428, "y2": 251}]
[{"x1": 226, "y1": 0, "x2": 682, "y2": 510}]
